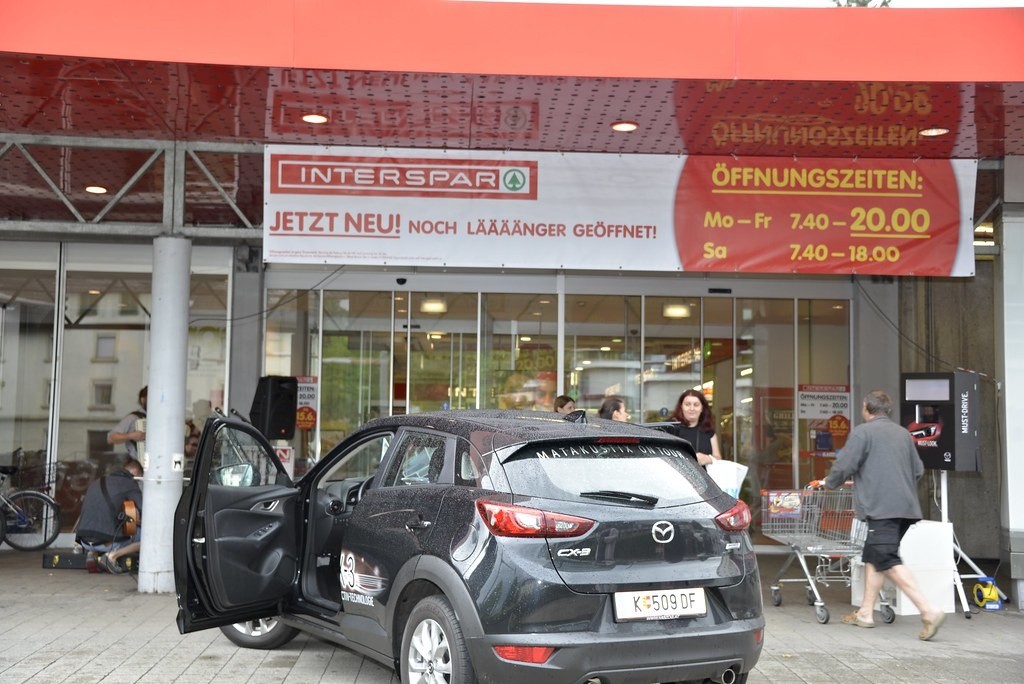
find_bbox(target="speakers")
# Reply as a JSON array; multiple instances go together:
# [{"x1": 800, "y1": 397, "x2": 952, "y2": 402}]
[
  {"x1": 900, "y1": 372, "x2": 982, "y2": 472},
  {"x1": 250, "y1": 376, "x2": 298, "y2": 441}
]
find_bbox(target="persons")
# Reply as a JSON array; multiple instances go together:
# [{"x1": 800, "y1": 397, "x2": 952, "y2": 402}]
[
  {"x1": 598, "y1": 399, "x2": 629, "y2": 422},
  {"x1": 75, "y1": 460, "x2": 143, "y2": 574},
  {"x1": 107, "y1": 385, "x2": 148, "y2": 460},
  {"x1": 673, "y1": 389, "x2": 722, "y2": 471},
  {"x1": 824, "y1": 391, "x2": 946, "y2": 641},
  {"x1": 556, "y1": 395, "x2": 575, "y2": 414}
]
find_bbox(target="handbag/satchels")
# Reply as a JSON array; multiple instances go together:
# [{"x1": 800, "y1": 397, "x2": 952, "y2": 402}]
[{"x1": 705, "y1": 454, "x2": 749, "y2": 498}]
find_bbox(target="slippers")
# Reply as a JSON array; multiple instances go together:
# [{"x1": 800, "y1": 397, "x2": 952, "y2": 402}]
[
  {"x1": 919, "y1": 611, "x2": 946, "y2": 640},
  {"x1": 842, "y1": 613, "x2": 875, "y2": 627}
]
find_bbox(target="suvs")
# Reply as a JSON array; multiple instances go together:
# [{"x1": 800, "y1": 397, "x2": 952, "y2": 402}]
[{"x1": 169, "y1": 405, "x2": 768, "y2": 684}]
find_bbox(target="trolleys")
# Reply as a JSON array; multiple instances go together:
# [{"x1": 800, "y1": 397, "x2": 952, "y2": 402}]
[{"x1": 760, "y1": 477, "x2": 895, "y2": 622}]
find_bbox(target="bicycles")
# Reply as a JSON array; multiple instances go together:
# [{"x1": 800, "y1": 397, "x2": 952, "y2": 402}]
[{"x1": 0, "y1": 465, "x2": 67, "y2": 551}]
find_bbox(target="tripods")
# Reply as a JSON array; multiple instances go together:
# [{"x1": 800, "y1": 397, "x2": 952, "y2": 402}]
[{"x1": 939, "y1": 471, "x2": 1011, "y2": 619}]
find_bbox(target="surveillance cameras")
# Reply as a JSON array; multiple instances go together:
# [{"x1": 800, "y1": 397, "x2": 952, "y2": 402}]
[{"x1": 397, "y1": 279, "x2": 406, "y2": 285}]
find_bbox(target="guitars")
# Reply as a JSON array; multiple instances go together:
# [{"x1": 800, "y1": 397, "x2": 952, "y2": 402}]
[{"x1": 121, "y1": 500, "x2": 138, "y2": 535}]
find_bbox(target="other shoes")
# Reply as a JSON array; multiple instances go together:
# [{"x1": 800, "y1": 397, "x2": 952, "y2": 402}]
[
  {"x1": 86, "y1": 552, "x2": 101, "y2": 573},
  {"x1": 99, "y1": 555, "x2": 123, "y2": 574}
]
